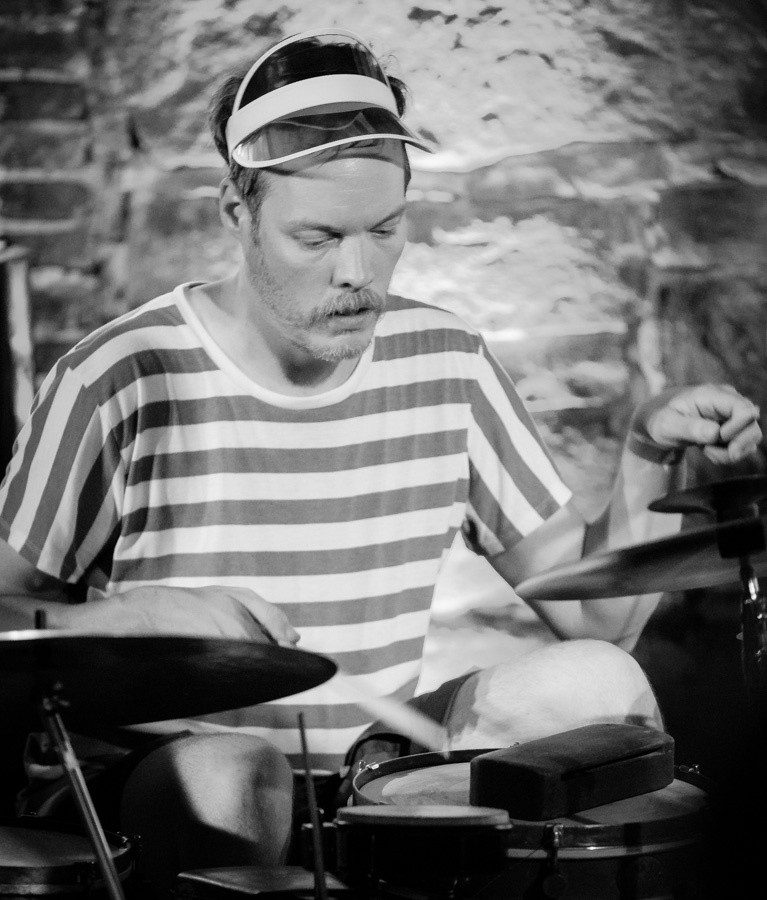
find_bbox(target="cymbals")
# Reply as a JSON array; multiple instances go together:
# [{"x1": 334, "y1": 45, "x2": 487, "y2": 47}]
[
  {"x1": 649, "y1": 475, "x2": 767, "y2": 514},
  {"x1": 515, "y1": 516, "x2": 767, "y2": 602},
  {"x1": 0, "y1": 635, "x2": 337, "y2": 735}
]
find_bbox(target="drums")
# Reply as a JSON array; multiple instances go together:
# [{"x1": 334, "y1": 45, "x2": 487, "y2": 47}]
[
  {"x1": 334, "y1": 803, "x2": 513, "y2": 900},
  {"x1": 0, "y1": 812, "x2": 134, "y2": 900},
  {"x1": 352, "y1": 747, "x2": 719, "y2": 900}
]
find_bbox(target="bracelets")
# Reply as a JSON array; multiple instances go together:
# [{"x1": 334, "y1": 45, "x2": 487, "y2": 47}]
[{"x1": 624, "y1": 434, "x2": 691, "y2": 466}]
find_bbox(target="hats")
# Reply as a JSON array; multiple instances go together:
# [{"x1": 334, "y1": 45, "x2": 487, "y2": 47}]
[{"x1": 225, "y1": 28, "x2": 437, "y2": 167}]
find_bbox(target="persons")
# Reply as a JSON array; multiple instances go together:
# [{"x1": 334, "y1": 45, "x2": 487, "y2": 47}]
[{"x1": 0, "y1": 30, "x2": 763, "y2": 888}]
